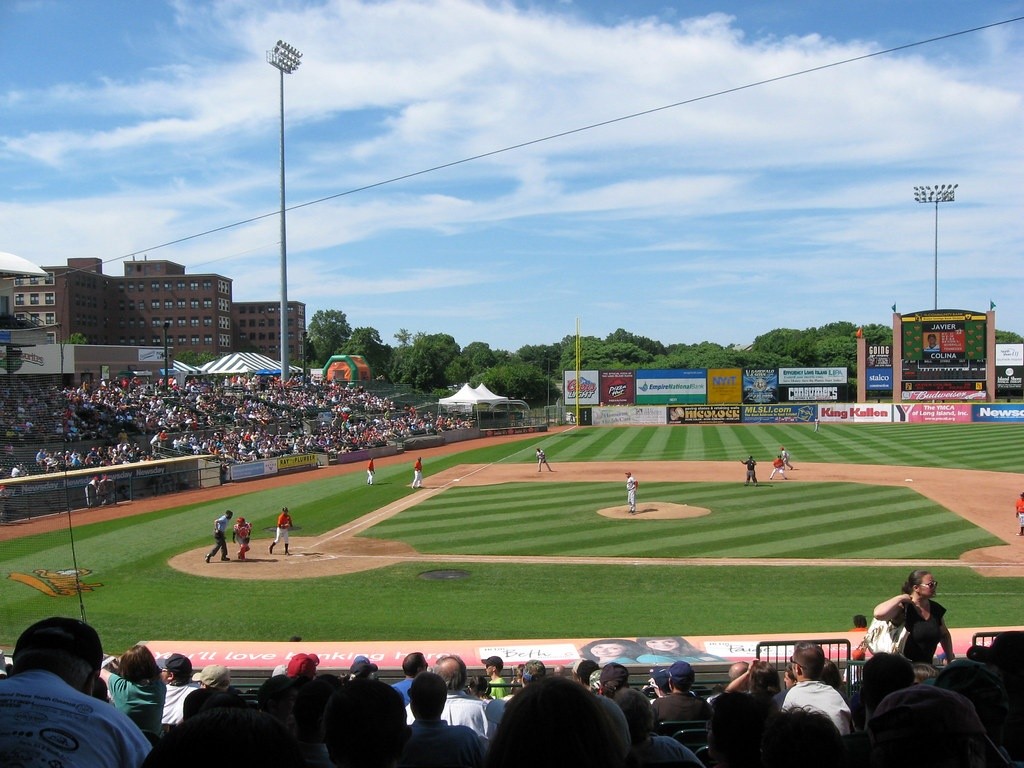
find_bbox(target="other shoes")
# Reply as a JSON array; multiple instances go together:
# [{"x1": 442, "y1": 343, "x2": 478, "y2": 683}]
[
  {"x1": 220, "y1": 556, "x2": 231, "y2": 561},
  {"x1": 269, "y1": 547, "x2": 273, "y2": 554},
  {"x1": 238, "y1": 556, "x2": 248, "y2": 561},
  {"x1": 206, "y1": 557, "x2": 210, "y2": 563},
  {"x1": 285, "y1": 551, "x2": 293, "y2": 555}
]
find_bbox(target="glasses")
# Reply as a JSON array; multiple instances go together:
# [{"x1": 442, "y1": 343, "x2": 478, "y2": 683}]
[
  {"x1": 422, "y1": 663, "x2": 428, "y2": 669},
  {"x1": 486, "y1": 664, "x2": 493, "y2": 669},
  {"x1": 920, "y1": 581, "x2": 938, "y2": 589},
  {"x1": 789, "y1": 657, "x2": 804, "y2": 669}
]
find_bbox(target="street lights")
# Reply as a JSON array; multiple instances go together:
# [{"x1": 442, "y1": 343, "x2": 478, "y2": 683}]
[
  {"x1": 163, "y1": 322, "x2": 170, "y2": 391},
  {"x1": 265, "y1": 39, "x2": 304, "y2": 383},
  {"x1": 912, "y1": 184, "x2": 959, "y2": 311}
]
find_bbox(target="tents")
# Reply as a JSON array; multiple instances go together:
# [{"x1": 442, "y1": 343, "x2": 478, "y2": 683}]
[{"x1": 438, "y1": 383, "x2": 510, "y2": 415}]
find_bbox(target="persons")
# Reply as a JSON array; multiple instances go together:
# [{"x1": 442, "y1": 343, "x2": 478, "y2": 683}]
[
  {"x1": 625, "y1": 472, "x2": 638, "y2": 513},
  {"x1": 569, "y1": 414, "x2": 575, "y2": 421},
  {"x1": 581, "y1": 640, "x2": 640, "y2": 669},
  {"x1": 0, "y1": 613, "x2": 1024, "y2": 768},
  {"x1": 739, "y1": 455, "x2": 758, "y2": 487},
  {"x1": 411, "y1": 456, "x2": 423, "y2": 489},
  {"x1": 269, "y1": 507, "x2": 293, "y2": 555},
  {"x1": 814, "y1": 416, "x2": 819, "y2": 432},
  {"x1": 926, "y1": 334, "x2": 939, "y2": 349},
  {"x1": 0, "y1": 376, "x2": 473, "y2": 522},
  {"x1": 205, "y1": 510, "x2": 233, "y2": 563},
  {"x1": 873, "y1": 571, "x2": 954, "y2": 664},
  {"x1": 770, "y1": 447, "x2": 793, "y2": 480},
  {"x1": 1016, "y1": 492, "x2": 1024, "y2": 536},
  {"x1": 535, "y1": 447, "x2": 552, "y2": 472},
  {"x1": 637, "y1": 636, "x2": 727, "y2": 665},
  {"x1": 232, "y1": 517, "x2": 252, "y2": 561},
  {"x1": 367, "y1": 456, "x2": 375, "y2": 485}
]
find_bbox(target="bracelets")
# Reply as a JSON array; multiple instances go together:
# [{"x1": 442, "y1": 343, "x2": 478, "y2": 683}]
[{"x1": 946, "y1": 654, "x2": 955, "y2": 660}]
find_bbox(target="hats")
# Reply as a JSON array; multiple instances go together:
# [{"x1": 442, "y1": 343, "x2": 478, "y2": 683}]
[
  {"x1": 485, "y1": 693, "x2": 517, "y2": 724},
  {"x1": 666, "y1": 661, "x2": 694, "y2": 684},
  {"x1": 350, "y1": 663, "x2": 378, "y2": 679},
  {"x1": 650, "y1": 666, "x2": 671, "y2": 693},
  {"x1": 868, "y1": 683, "x2": 1010, "y2": 768},
  {"x1": 14, "y1": 617, "x2": 103, "y2": 674},
  {"x1": 237, "y1": 517, "x2": 245, "y2": 523},
  {"x1": 157, "y1": 653, "x2": 192, "y2": 679},
  {"x1": 191, "y1": 665, "x2": 231, "y2": 688},
  {"x1": 967, "y1": 631, "x2": 1024, "y2": 674},
  {"x1": 258, "y1": 674, "x2": 308, "y2": 707},
  {"x1": 272, "y1": 665, "x2": 288, "y2": 678},
  {"x1": 600, "y1": 662, "x2": 629, "y2": 690},
  {"x1": 354, "y1": 657, "x2": 370, "y2": 665},
  {"x1": 282, "y1": 507, "x2": 289, "y2": 512},
  {"x1": 596, "y1": 693, "x2": 632, "y2": 758},
  {"x1": 288, "y1": 653, "x2": 319, "y2": 678},
  {"x1": 481, "y1": 655, "x2": 503, "y2": 669}
]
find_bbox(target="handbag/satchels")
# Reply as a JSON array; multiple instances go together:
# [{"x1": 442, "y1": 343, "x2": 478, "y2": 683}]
[{"x1": 864, "y1": 598, "x2": 910, "y2": 654}]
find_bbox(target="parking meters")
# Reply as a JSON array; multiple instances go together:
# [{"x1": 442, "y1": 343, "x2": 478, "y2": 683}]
[{"x1": 543, "y1": 350, "x2": 550, "y2": 407}]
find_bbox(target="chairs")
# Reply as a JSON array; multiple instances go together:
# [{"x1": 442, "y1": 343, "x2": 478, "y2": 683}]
[
  {"x1": 103, "y1": 639, "x2": 970, "y2": 768},
  {"x1": 1, "y1": 378, "x2": 479, "y2": 527}
]
